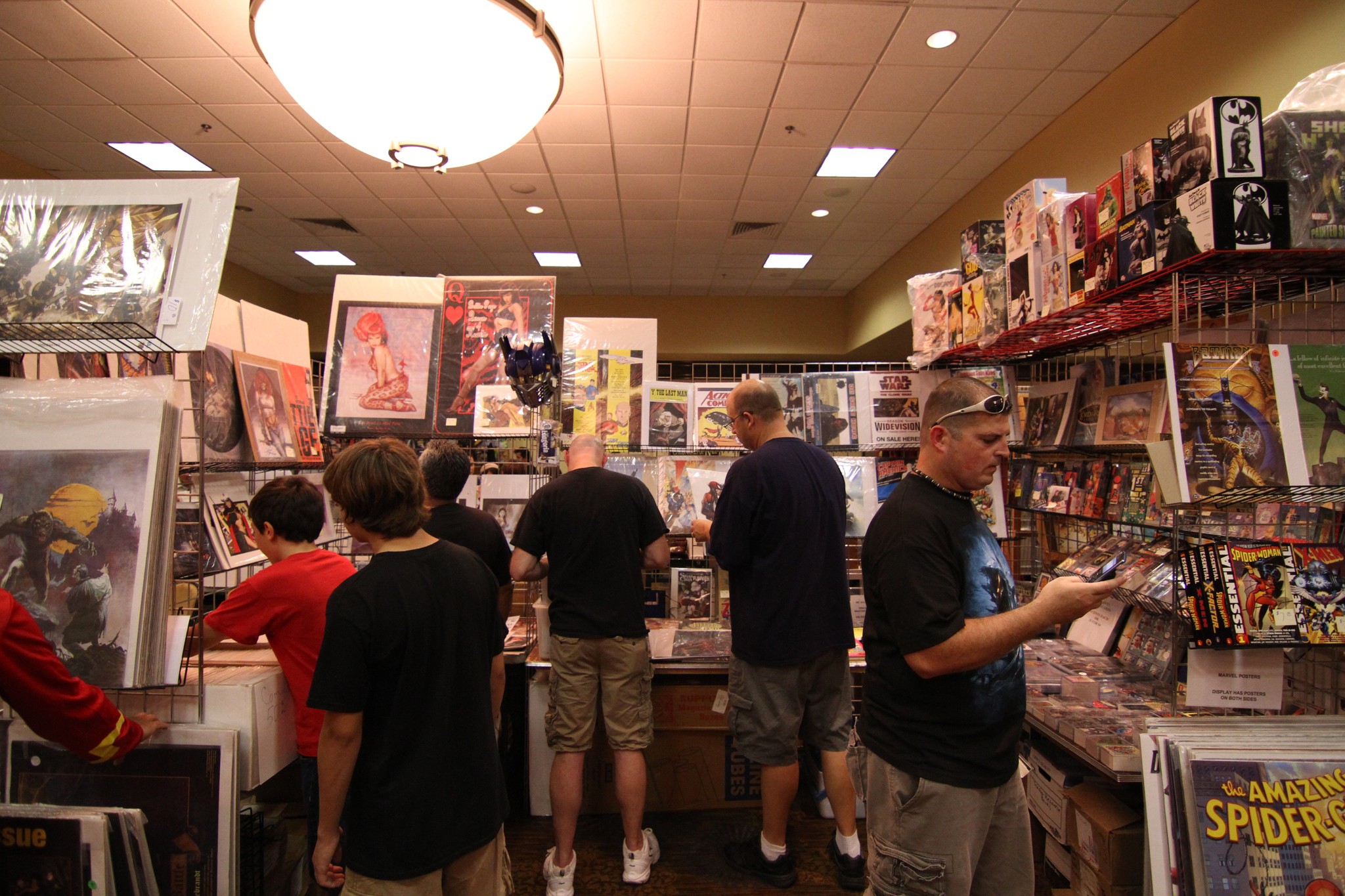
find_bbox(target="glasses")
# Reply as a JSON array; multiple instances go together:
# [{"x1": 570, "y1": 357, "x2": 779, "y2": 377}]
[
  {"x1": 929, "y1": 394, "x2": 1013, "y2": 430},
  {"x1": 724, "y1": 411, "x2": 753, "y2": 431}
]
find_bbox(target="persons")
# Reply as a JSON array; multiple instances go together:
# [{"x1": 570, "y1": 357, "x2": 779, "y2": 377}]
[
  {"x1": 305, "y1": 435, "x2": 516, "y2": 896},
  {"x1": 420, "y1": 439, "x2": 514, "y2": 620},
  {"x1": 0, "y1": 586, "x2": 167, "y2": 763},
  {"x1": 354, "y1": 311, "x2": 416, "y2": 411},
  {"x1": 183, "y1": 475, "x2": 363, "y2": 850},
  {"x1": 508, "y1": 433, "x2": 674, "y2": 896},
  {"x1": 445, "y1": 282, "x2": 526, "y2": 415},
  {"x1": 465, "y1": 440, "x2": 562, "y2": 481},
  {"x1": 855, "y1": 376, "x2": 1127, "y2": 896},
  {"x1": 687, "y1": 376, "x2": 867, "y2": 891}
]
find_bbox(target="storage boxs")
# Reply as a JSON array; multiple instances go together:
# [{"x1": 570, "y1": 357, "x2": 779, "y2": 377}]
[
  {"x1": 1026, "y1": 746, "x2": 1146, "y2": 896},
  {"x1": 579, "y1": 682, "x2": 764, "y2": 811},
  {"x1": 947, "y1": 94, "x2": 1345, "y2": 348},
  {"x1": 1009, "y1": 457, "x2": 1197, "y2": 529}
]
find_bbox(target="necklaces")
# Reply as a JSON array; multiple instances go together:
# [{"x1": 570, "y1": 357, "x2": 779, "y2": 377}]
[{"x1": 909, "y1": 464, "x2": 974, "y2": 500}]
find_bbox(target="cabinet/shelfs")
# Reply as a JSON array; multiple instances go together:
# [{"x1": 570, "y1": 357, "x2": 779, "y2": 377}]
[{"x1": 905, "y1": 247, "x2": 1344, "y2": 896}]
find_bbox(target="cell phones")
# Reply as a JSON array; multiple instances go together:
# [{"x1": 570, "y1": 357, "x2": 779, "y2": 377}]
[{"x1": 1084, "y1": 551, "x2": 1126, "y2": 584}]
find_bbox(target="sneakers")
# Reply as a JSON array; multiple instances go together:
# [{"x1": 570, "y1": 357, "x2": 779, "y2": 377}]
[
  {"x1": 621, "y1": 830, "x2": 661, "y2": 884},
  {"x1": 722, "y1": 835, "x2": 796, "y2": 889},
  {"x1": 543, "y1": 847, "x2": 576, "y2": 896},
  {"x1": 830, "y1": 830, "x2": 868, "y2": 890}
]
